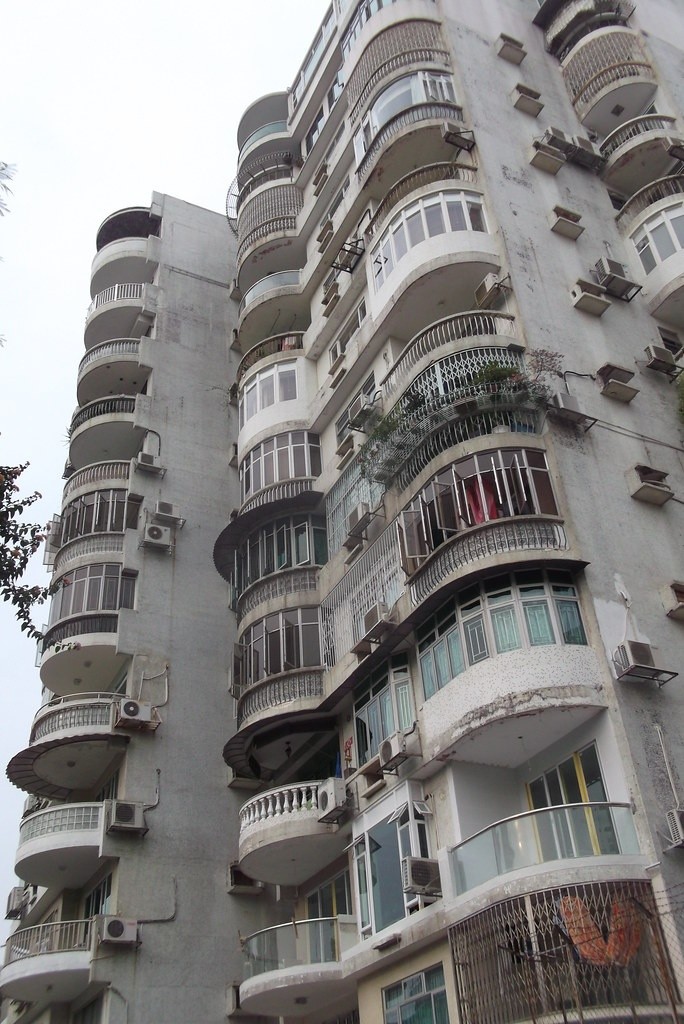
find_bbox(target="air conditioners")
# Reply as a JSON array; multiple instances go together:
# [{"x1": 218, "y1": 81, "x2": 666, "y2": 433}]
[
  {"x1": 400, "y1": 856, "x2": 442, "y2": 894},
  {"x1": 545, "y1": 126, "x2": 684, "y2": 422},
  {"x1": 64, "y1": 458, "x2": 72, "y2": 477},
  {"x1": 347, "y1": 394, "x2": 370, "y2": 424},
  {"x1": 6, "y1": 886, "x2": 23, "y2": 918},
  {"x1": 473, "y1": 273, "x2": 499, "y2": 303},
  {"x1": 107, "y1": 802, "x2": 144, "y2": 834},
  {"x1": 143, "y1": 500, "x2": 180, "y2": 549},
  {"x1": 99, "y1": 917, "x2": 138, "y2": 944},
  {"x1": 337, "y1": 237, "x2": 357, "y2": 267},
  {"x1": 348, "y1": 501, "x2": 389, "y2": 633},
  {"x1": 318, "y1": 778, "x2": 346, "y2": 822},
  {"x1": 439, "y1": 122, "x2": 471, "y2": 146},
  {"x1": 666, "y1": 809, "x2": 684, "y2": 842},
  {"x1": 117, "y1": 698, "x2": 151, "y2": 723},
  {"x1": 71, "y1": 406, "x2": 79, "y2": 420},
  {"x1": 618, "y1": 639, "x2": 655, "y2": 672},
  {"x1": 137, "y1": 451, "x2": 162, "y2": 472},
  {"x1": 376, "y1": 732, "x2": 407, "y2": 767},
  {"x1": 23, "y1": 795, "x2": 34, "y2": 817}
]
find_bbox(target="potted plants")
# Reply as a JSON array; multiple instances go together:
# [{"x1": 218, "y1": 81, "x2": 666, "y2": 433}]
[{"x1": 357, "y1": 364, "x2": 529, "y2": 484}]
